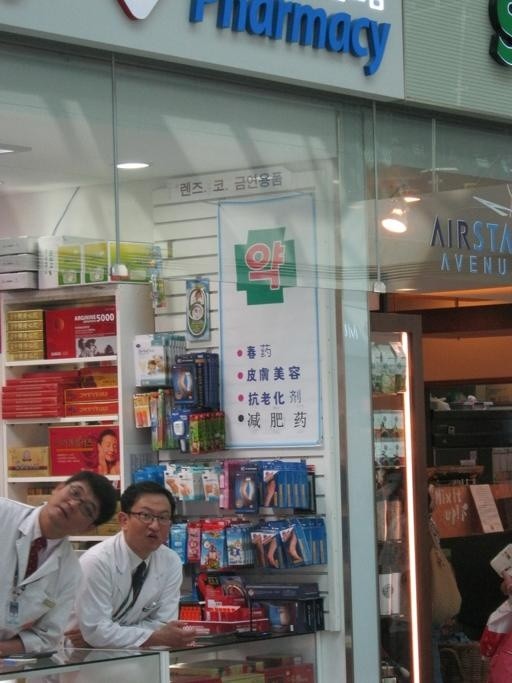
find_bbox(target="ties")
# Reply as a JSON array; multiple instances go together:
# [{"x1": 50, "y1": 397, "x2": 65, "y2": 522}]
[
  {"x1": 23, "y1": 538, "x2": 47, "y2": 580},
  {"x1": 132, "y1": 561, "x2": 147, "y2": 598}
]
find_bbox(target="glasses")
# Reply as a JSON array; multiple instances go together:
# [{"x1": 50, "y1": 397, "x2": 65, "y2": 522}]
[
  {"x1": 131, "y1": 511, "x2": 173, "y2": 527},
  {"x1": 69, "y1": 484, "x2": 95, "y2": 520}
]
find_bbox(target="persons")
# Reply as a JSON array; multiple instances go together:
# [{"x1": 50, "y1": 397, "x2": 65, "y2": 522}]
[
  {"x1": 0, "y1": 470, "x2": 118, "y2": 657},
  {"x1": 96, "y1": 429, "x2": 119, "y2": 475},
  {"x1": 63, "y1": 479, "x2": 197, "y2": 649},
  {"x1": 478, "y1": 570, "x2": 512, "y2": 683},
  {"x1": 378, "y1": 479, "x2": 444, "y2": 683}
]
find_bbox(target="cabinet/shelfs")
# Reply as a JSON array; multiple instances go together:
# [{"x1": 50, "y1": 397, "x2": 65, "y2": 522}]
[
  {"x1": 0, "y1": 284, "x2": 156, "y2": 555},
  {"x1": 427, "y1": 376, "x2": 512, "y2": 531}
]
